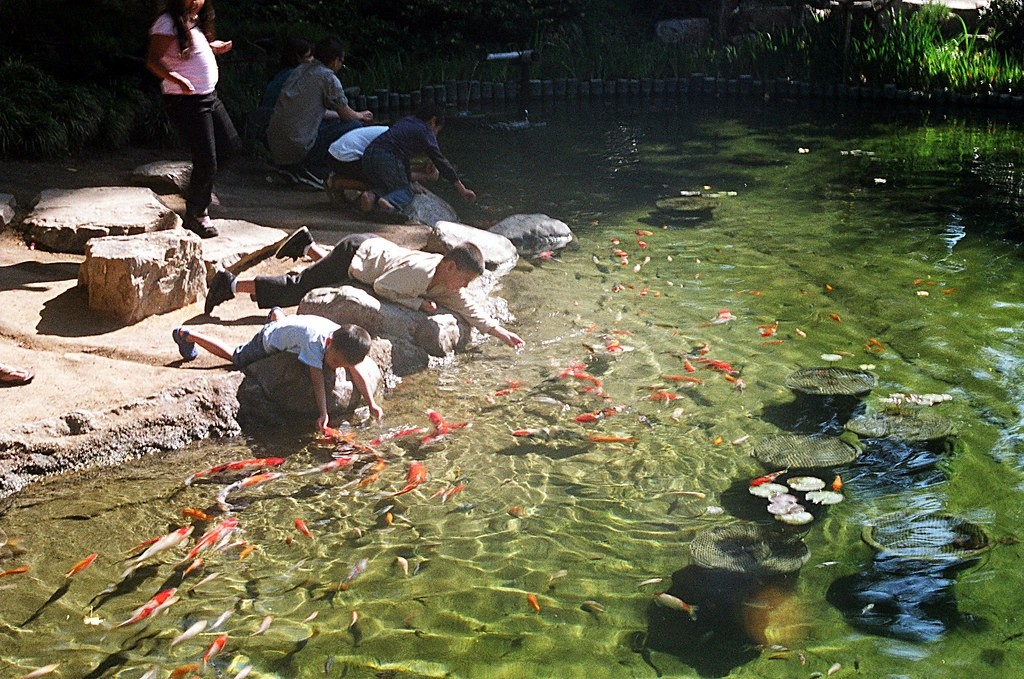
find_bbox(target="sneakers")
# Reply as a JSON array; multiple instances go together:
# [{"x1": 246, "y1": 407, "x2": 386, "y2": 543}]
[
  {"x1": 205, "y1": 270, "x2": 236, "y2": 319},
  {"x1": 275, "y1": 226, "x2": 314, "y2": 265}
]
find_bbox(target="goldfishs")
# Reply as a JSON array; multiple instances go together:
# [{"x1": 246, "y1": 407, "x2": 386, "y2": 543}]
[{"x1": 0, "y1": 220, "x2": 954, "y2": 679}]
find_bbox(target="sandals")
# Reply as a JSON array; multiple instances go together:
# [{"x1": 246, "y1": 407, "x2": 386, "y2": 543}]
[
  {"x1": 0, "y1": 363, "x2": 34, "y2": 387},
  {"x1": 267, "y1": 308, "x2": 283, "y2": 326},
  {"x1": 173, "y1": 327, "x2": 198, "y2": 360}
]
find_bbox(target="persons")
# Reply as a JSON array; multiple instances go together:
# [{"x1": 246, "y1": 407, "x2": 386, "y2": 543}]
[
  {"x1": 172, "y1": 307, "x2": 382, "y2": 430},
  {"x1": 0, "y1": 363, "x2": 35, "y2": 387},
  {"x1": 147, "y1": 0, "x2": 243, "y2": 239},
  {"x1": 205, "y1": 226, "x2": 526, "y2": 350},
  {"x1": 327, "y1": 104, "x2": 475, "y2": 212},
  {"x1": 264, "y1": 38, "x2": 373, "y2": 190}
]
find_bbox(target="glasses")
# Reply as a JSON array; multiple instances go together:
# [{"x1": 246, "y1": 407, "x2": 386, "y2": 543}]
[{"x1": 339, "y1": 57, "x2": 346, "y2": 69}]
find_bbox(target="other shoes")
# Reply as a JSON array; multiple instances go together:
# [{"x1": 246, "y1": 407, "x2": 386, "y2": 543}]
[
  {"x1": 297, "y1": 169, "x2": 326, "y2": 190},
  {"x1": 182, "y1": 215, "x2": 217, "y2": 238}
]
